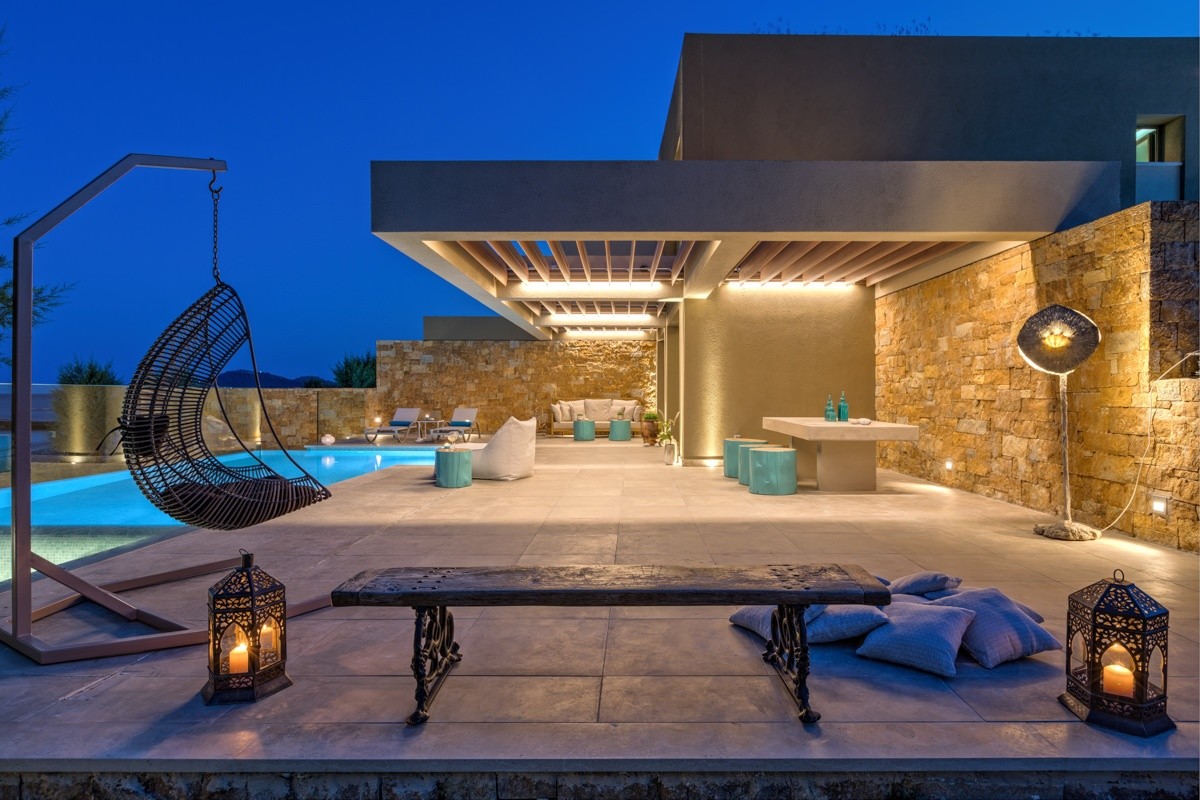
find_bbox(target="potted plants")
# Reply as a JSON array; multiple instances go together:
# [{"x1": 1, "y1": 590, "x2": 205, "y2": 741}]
[
  {"x1": 640, "y1": 412, "x2": 659, "y2": 446},
  {"x1": 617, "y1": 411, "x2": 624, "y2": 420}
]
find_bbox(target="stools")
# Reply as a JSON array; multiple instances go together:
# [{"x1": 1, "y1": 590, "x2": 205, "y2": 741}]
[
  {"x1": 738, "y1": 443, "x2": 786, "y2": 486},
  {"x1": 435, "y1": 448, "x2": 472, "y2": 488},
  {"x1": 574, "y1": 419, "x2": 596, "y2": 442},
  {"x1": 608, "y1": 419, "x2": 631, "y2": 441},
  {"x1": 200, "y1": 552, "x2": 294, "y2": 708},
  {"x1": 723, "y1": 438, "x2": 769, "y2": 478},
  {"x1": 749, "y1": 448, "x2": 797, "y2": 494}
]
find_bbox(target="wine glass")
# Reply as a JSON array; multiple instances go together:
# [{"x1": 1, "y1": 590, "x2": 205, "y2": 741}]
[{"x1": 448, "y1": 431, "x2": 459, "y2": 451}]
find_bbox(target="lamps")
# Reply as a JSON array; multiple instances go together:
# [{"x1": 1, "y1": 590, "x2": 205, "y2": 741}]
[
  {"x1": 1056, "y1": 568, "x2": 1177, "y2": 738},
  {"x1": 1016, "y1": 304, "x2": 1104, "y2": 541}
]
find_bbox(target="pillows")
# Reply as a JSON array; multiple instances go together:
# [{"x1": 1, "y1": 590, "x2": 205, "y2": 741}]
[
  {"x1": 729, "y1": 569, "x2": 1063, "y2": 679},
  {"x1": 550, "y1": 398, "x2": 638, "y2": 420}
]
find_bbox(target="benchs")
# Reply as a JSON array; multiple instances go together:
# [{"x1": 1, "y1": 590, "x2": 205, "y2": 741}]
[
  {"x1": 331, "y1": 563, "x2": 890, "y2": 726},
  {"x1": 550, "y1": 413, "x2": 643, "y2": 438}
]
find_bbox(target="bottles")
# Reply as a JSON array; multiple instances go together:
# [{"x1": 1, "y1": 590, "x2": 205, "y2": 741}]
[
  {"x1": 825, "y1": 394, "x2": 836, "y2": 421},
  {"x1": 838, "y1": 388, "x2": 848, "y2": 421}
]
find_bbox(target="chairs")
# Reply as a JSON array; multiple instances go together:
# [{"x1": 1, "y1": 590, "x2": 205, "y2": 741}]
[
  {"x1": 364, "y1": 407, "x2": 420, "y2": 443},
  {"x1": 448, "y1": 416, "x2": 536, "y2": 482},
  {"x1": 431, "y1": 408, "x2": 481, "y2": 443}
]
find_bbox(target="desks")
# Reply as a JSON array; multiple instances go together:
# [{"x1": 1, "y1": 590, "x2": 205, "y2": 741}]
[
  {"x1": 416, "y1": 420, "x2": 444, "y2": 443},
  {"x1": 762, "y1": 416, "x2": 919, "y2": 491}
]
